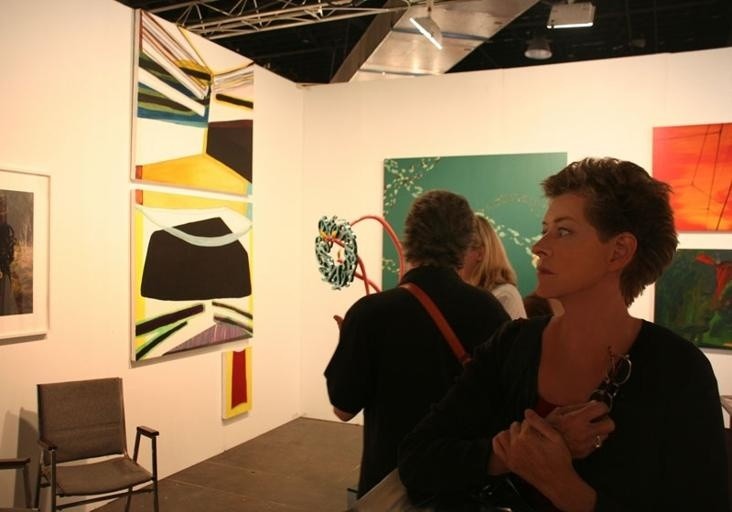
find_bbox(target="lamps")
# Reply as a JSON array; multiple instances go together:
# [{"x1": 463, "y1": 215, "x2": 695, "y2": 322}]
[
  {"x1": 409, "y1": 1, "x2": 444, "y2": 49},
  {"x1": 523, "y1": 28, "x2": 553, "y2": 59},
  {"x1": 547, "y1": 0, "x2": 596, "y2": 29}
]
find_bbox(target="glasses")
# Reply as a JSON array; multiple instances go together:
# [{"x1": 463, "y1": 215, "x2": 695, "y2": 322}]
[{"x1": 588, "y1": 343, "x2": 634, "y2": 415}]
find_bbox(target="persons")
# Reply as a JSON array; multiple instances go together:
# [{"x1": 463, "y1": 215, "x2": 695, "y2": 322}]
[
  {"x1": 457, "y1": 214, "x2": 529, "y2": 321},
  {"x1": 399, "y1": 156, "x2": 730, "y2": 511},
  {"x1": 322, "y1": 190, "x2": 512, "y2": 499}
]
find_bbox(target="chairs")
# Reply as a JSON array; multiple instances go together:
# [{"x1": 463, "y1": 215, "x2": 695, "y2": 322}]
[
  {"x1": 35, "y1": 377, "x2": 160, "y2": 511},
  {"x1": 1, "y1": 456, "x2": 39, "y2": 512}
]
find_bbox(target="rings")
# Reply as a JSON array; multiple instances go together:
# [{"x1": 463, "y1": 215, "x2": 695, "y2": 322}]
[{"x1": 591, "y1": 431, "x2": 601, "y2": 448}]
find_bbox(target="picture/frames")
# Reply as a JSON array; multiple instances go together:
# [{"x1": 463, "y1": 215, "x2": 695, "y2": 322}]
[{"x1": 0, "y1": 168, "x2": 50, "y2": 341}]
